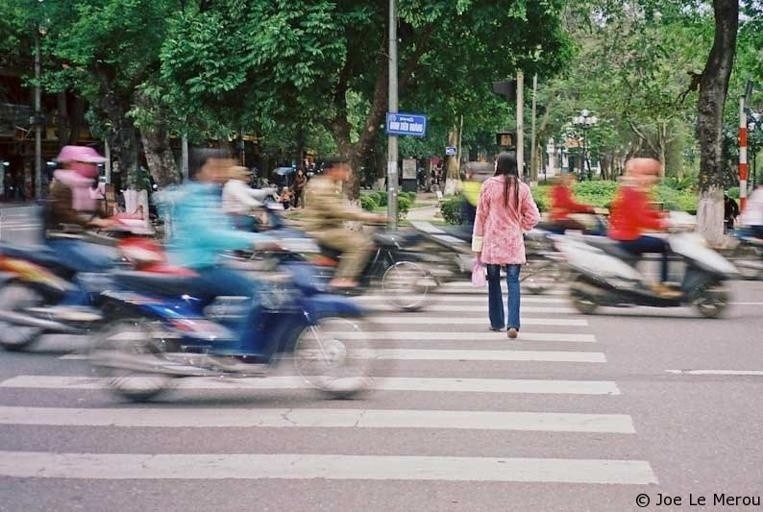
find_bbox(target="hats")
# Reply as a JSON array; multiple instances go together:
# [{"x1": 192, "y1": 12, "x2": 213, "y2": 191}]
[
  {"x1": 52, "y1": 145, "x2": 109, "y2": 166},
  {"x1": 227, "y1": 166, "x2": 253, "y2": 179}
]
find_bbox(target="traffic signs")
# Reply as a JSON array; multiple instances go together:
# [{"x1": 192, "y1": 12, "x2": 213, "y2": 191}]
[{"x1": 384, "y1": 111, "x2": 426, "y2": 137}]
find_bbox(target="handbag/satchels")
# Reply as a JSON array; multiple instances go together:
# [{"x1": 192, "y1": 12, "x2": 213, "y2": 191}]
[{"x1": 470, "y1": 260, "x2": 488, "y2": 287}]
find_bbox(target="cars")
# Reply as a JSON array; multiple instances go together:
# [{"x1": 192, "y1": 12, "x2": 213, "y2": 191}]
[{"x1": 459, "y1": 161, "x2": 493, "y2": 182}]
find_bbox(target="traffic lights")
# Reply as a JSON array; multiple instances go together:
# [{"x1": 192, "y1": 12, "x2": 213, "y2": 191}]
[
  {"x1": 496, "y1": 132, "x2": 514, "y2": 146},
  {"x1": 491, "y1": 79, "x2": 516, "y2": 100}
]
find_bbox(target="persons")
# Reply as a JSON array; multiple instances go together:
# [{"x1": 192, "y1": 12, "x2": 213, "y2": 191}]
[
  {"x1": 15, "y1": 169, "x2": 28, "y2": 203},
  {"x1": 159, "y1": 147, "x2": 282, "y2": 374},
  {"x1": 44, "y1": 144, "x2": 128, "y2": 321},
  {"x1": 607, "y1": 156, "x2": 684, "y2": 299},
  {"x1": 289, "y1": 168, "x2": 308, "y2": 209},
  {"x1": 416, "y1": 158, "x2": 445, "y2": 190},
  {"x1": 3, "y1": 172, "x2": 13, "y2": 203},
  {"x1": 547, "y1": 172, "x2": 607, "y2": 232},
  {"x1": 295, "y1": 153, "x2": 397, "y2": 290},
  {"x1": 471, "y1": 152, "x2": 541, "y2": 339},
  {"x1": 724, "y1": 194, "x2": 739, "y2": 230},
  {"x1": 221, "y1": 164, "x2": 279, "y2": 232},
  {"x1": 279, "y1": 187, "x2": 293, "y2": 210},
  {"x1": 737, "y1": 173, "x2": 763, "y2": 239}
]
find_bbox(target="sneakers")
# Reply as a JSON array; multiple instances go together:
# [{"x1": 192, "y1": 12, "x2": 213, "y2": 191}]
[
  {"x1": 653, "y1": 283, "x2": 682, "y2": 298},
  {"x1": 507, "y1": 327, "x2": 517, "y2": 337}
]
